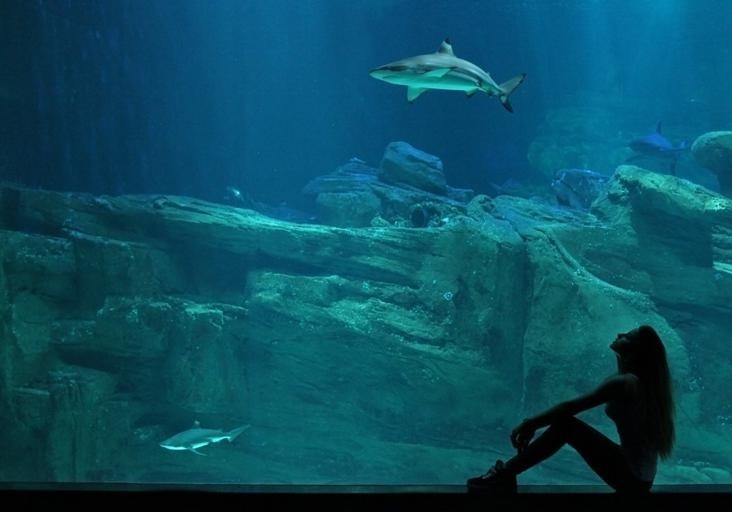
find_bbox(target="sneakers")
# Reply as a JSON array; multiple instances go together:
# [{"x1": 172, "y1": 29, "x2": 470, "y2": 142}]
[{"x1": 466, "y1": 459, "x2": 517, "y2": 489}]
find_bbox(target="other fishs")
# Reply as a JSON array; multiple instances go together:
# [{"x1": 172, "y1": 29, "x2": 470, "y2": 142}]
[{"x1": 624, "y1": 121, "x2": 689, "y2": 172}]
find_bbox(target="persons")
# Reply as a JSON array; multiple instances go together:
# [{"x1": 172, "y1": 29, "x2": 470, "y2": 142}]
[{"x1": 465, "y1": 325, "x2": 676, "y2": 493}]
